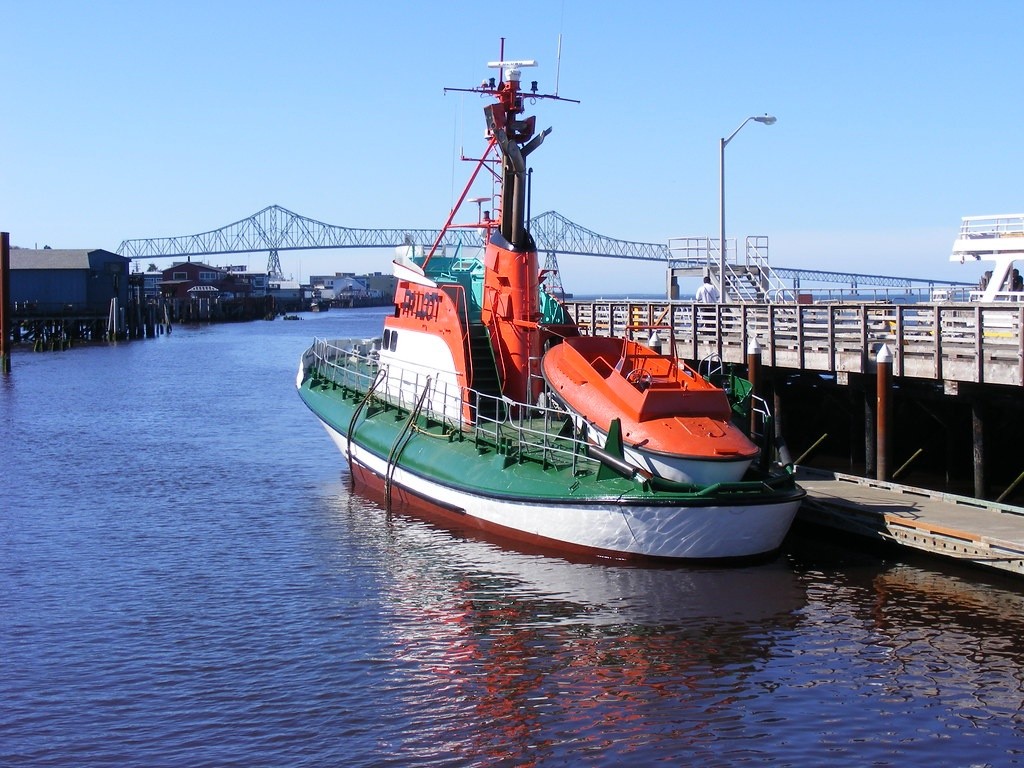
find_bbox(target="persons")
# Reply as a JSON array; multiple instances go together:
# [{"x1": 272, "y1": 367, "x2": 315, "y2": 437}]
[
  {"x1": 695, "y1": 277, "x2": 719, "y2": 336},
  {"x1": 1012, "y1": 269, "x2": 1024, "y2": 301}
]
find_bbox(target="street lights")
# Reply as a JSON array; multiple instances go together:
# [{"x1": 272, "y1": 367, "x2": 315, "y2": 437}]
[{"x1": 718, "y1": 112, "x2": 778, "y2": 333}]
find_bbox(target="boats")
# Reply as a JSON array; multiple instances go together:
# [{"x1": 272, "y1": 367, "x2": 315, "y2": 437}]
[
  {"x1": 296, "y1": 33, "x2": 811, "y2": 572},
  {"x1": 311, "y1": 297, "x2": 329, "y2": 312},
  {"x1": 535, "y1": 334, "x2": 762, "y2": 490}
]
